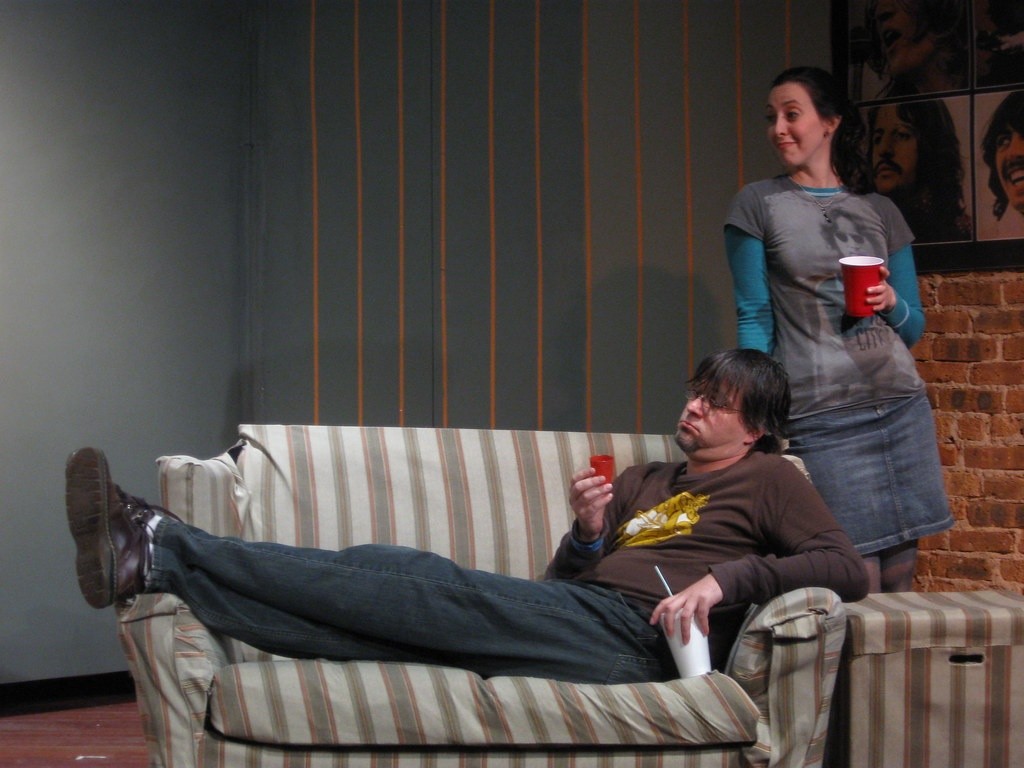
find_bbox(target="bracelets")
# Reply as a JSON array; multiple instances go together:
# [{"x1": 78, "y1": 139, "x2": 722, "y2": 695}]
[
  {"x1": 891, "y1": 297, "x2": 910, "y2": 329},
  {"x1": 569, "y1": 528, "x2": 605, "y2": 553}
]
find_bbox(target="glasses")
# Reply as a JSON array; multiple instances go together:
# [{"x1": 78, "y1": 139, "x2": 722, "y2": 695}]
[{"x1": 681, "y1": 388, "x2": 741, "y2": 413}]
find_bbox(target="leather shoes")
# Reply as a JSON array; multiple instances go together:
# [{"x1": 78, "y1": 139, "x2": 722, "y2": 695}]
[{"x1": 65, "y1": 447, "x2": 155, "y2": 609}]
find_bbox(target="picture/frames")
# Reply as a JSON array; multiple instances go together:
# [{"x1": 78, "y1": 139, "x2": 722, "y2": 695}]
[{"x1": 829, "y1": 0, "x2": 1024, "y2": 273}]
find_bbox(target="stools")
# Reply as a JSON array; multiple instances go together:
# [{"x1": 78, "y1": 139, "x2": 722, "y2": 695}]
[{"x1": 824, "y1": 586, "x2": 1024, "y2": 768}]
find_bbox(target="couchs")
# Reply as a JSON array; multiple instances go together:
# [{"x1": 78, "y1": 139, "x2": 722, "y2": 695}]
[{"x1": 118, "y1": 430, "x2": 846, "y2": 768}]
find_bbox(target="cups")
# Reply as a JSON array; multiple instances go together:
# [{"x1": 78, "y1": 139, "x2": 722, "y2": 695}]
[
  {"x1": 589, "y1": 455, "x2": 615, "y2": 494},
  {"x1": 838, "y1": 255, "x2": 885, "y2": 319},
  {"x1": 660, "y1": 607, "x2": 712, "y2": 678}
]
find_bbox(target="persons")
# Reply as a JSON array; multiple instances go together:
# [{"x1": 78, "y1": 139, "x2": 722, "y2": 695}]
[
  {"x1": 847, "y1": 1, "x2": 1023, "y2": 241},
  {"x1": 63, "y1": 345, "x2": 871, "y2": 684},
  {"x1": 976, "y1": 1, "x2": 1024, "y2": 86},
  {"x1": 720, "y1": 65, "x2": 958, "y2": 595}
]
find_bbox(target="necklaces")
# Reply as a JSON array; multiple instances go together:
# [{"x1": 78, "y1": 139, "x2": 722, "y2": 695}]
[{"x1": 789, "y1": 171, "x2": 843, "y2": 224}]
